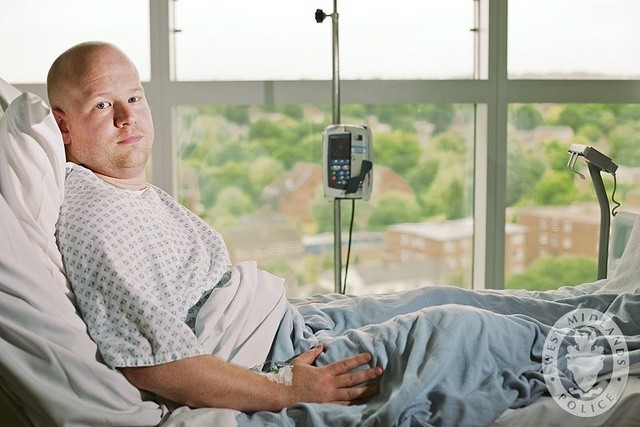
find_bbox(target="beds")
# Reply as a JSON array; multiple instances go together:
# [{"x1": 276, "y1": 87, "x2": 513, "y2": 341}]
[{"x1": 1, "y1": 74, "x2": 636, "y2": 427}]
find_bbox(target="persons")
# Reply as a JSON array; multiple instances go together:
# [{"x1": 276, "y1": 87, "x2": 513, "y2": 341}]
[{"x1": 46, "y1": 41, "x2": 640, "y2": 412}]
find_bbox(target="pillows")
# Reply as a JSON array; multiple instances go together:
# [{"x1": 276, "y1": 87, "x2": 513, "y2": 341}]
[{"x1": 0, "y1": 93, "x2": 71, "y2": 246}]
